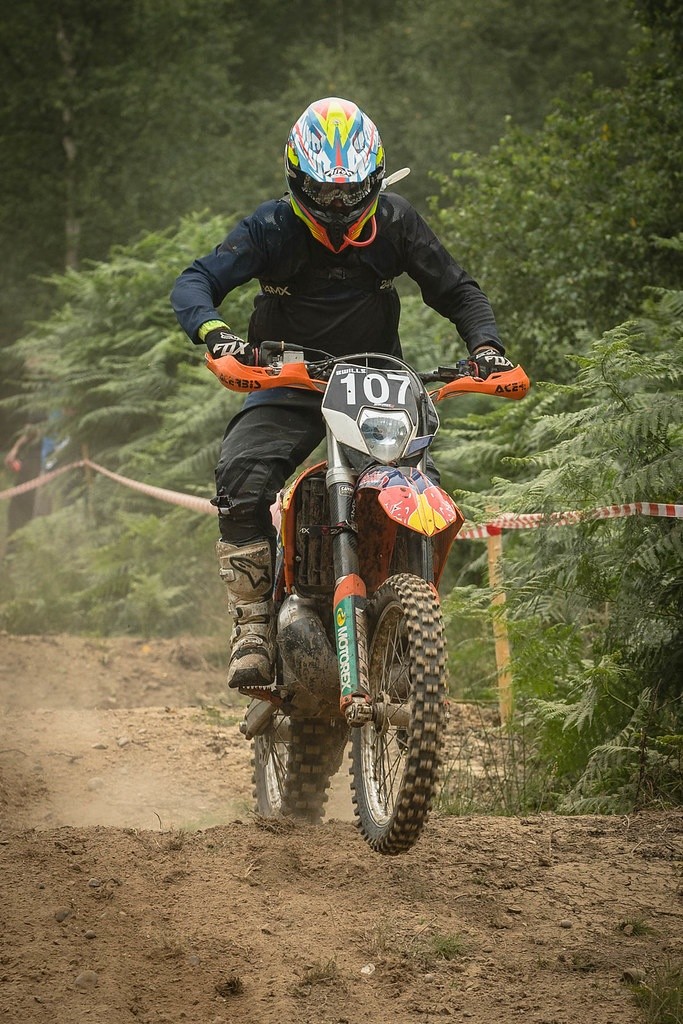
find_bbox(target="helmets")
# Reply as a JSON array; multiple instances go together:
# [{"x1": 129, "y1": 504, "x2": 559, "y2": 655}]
[{"x1": 285, "y1": 98, "x2": 385, "y2": 254}]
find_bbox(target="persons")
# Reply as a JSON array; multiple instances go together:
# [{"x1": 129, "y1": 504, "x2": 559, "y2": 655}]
[
  {"x1": 2, "y1": 402, "x2": 93, "y2": 533},
  {"x1": 172, "y1": 97, "x2": 517, "y2": 687}
]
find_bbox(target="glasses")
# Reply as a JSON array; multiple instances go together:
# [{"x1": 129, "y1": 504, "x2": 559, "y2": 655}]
[{"x1": 287, "y1": 158, "x2": 383, "y2": 213}]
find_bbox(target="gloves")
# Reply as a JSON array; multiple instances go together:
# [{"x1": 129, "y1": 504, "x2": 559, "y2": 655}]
[
  {"x1": 205, "y1": 327, "x2": 252, "y2": 364},
  {"x1": 469, "y1": 348, "x2": 514, "y2": 381}
]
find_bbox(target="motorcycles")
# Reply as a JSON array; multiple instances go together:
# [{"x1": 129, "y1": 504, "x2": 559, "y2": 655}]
[{"x1": 202, "y1": 340, "x2": 530, "y2": 856}]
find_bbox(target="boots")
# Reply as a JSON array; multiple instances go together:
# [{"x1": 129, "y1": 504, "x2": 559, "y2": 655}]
[{"x1": 214, "y1": 537, "x2": 278, "y2": 688}]
